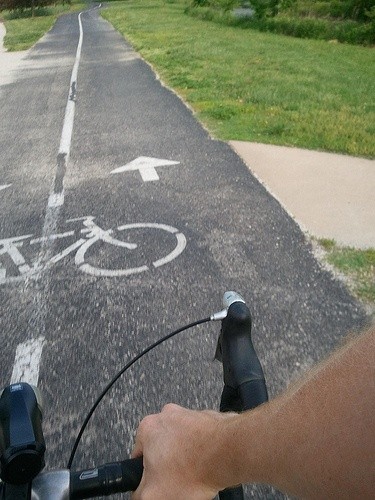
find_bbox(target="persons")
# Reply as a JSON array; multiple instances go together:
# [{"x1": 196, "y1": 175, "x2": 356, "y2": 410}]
[{"x1": 129, "y1": 314, "x2": 375, "y2": 499}]
